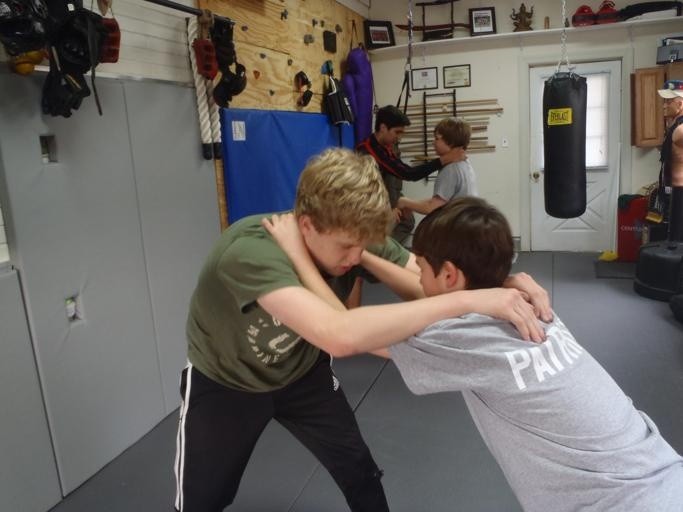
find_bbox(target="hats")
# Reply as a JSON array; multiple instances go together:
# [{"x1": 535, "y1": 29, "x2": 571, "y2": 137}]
[{"x1": 657, "y1": 80, "x2": 683, "y2": 100}]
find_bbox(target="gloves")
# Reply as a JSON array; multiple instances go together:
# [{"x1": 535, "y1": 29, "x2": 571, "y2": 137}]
[{"x1": 213, "y1": 64, "x2": 247, "y2": 108}]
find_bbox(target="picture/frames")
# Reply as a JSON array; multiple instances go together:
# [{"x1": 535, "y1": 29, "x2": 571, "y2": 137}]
[
  {"x1": 362, "y1": 18, "x2": 396, "y2": 51},
  {"x1": 467, "y1": 6, "x2": 496, "y2": 37},
  {"x1": 441, "y1": 64, "x2": 471, "y2": 89},
  {"x1": 410, "y1": 67, "x2": 438, "y2": 92}
]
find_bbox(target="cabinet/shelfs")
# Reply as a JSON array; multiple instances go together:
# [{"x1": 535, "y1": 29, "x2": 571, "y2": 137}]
[{"x1": 633, "y1": 59, "x2": 682, "y2": 149}]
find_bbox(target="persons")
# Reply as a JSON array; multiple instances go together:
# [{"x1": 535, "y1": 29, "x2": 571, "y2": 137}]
[
  {"x1": 396, "y1": 115, "x2": 474, "y2": 216},
  {"x1": 343, "y1": 104, "x2": 468, "y2": 310},
  {"x1": 172, "y1": 146, "x2": 555, "y2": 512},
  {"x1": 261, "y1": 195, "x2": 681, "y2": 512},
  {"x1": 657, "y1": 79, "x2": 682, "y2": 243}
]
